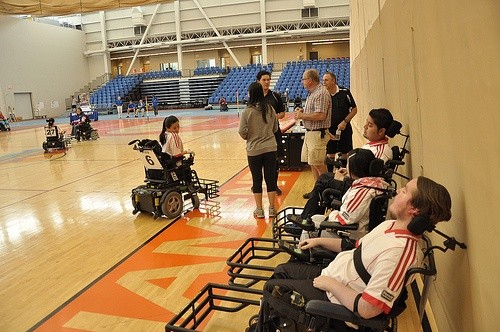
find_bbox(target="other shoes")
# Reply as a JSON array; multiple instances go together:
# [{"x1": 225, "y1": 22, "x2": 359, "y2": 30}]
[
  {"x1": 245, "y1": 315, "x2": 280, "y2": 332},
  {"x1": 303, "y1": 191, "x2": 312, "y2": 199},
  {"x1": 275, "y1": 186, "x2": 281, "y2": 195},
  {"x1": 269, "y1": 208, "x2": 277, "y2": 217},
  {"x1": 254, "y1": 209, "x2": 265, "y2": 217},
  {"x1": 287, "y1": 214, "x2": 302, "y2": 225}
]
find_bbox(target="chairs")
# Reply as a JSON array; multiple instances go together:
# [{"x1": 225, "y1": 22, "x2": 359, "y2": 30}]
[
  {"x1": 89, "y1": 69, "x2": 181, "y2": 105},
  {"x1": 273, "y1": 56, "x2": 350, "y2": 103},
  {"x1": 193, "y1": 63, "x2": 273, "y2": 103}
]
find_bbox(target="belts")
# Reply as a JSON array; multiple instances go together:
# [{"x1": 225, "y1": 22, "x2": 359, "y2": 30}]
[{"x1": 306, "y1": 128, "x2": 328, "y2": 131}]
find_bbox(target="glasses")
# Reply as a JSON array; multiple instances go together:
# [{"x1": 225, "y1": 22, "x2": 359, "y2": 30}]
[{"x1": 300, "y1": 77, "x2": 309, "y2": 82}]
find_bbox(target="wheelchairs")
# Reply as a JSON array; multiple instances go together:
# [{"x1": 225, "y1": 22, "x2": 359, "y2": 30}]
[
  {"x1": 71, "y1": 117, "x2": 99, "y2": 141},
  {"x1": 0, "y1": 119, "x2": 11, "y2": 132},
  {"x1": 164, "y1": 229, "x2": 468, "y2": 332},
  {"x1": 226, "y1": 145, "x2": 411, "y2": 289},
  {"x1": 42, "y1": 126, "x2": 71, "y2": 151},
  {"x1": 128, "y1": 139, "x2": 220, "y2": 219},
  {"x1": 219, "y1": 102, "x2": 229, "y2": 112}
]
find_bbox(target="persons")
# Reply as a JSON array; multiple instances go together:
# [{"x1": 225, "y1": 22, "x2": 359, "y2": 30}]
[
  {"x1": 158, "y1": 115, "x2": 201, "y2": 192},
  {"x1": 126, "y1": 99, "x2": 146, "y2": 118},
  {"x1": 0, "y1": 111, "x2": 10, "y2": 128},
  {"x1": 323, "y1": 71, "x2": 357, "y2": 174},
  {"x1": 45, "y1": 118, "x2": 66, "y2": 138},
  {"x1": 152, "y1": 94, "x2": 158, "y2": 116},
  {"x1": 237, "y1": 81, "x2": 278, "y2": 219},
  {"x1": 71, "y1": 96, "x2": 78, "y2": 112},
  {"x1": 283, "y1": 108, "x2": 402, "y2": 239},
  {"x1": 244, "y1": 176, "x2": 452, "y2": 332},
  {"x1": 256, "y1": 70, "x2": 286, "y2": 195},
  {"x1": 293, "y1": 68, "x2": 332, "y2": 199},
  {"x1": 115, "y1": 96, "x2": 123, "y2": 118},
  {"x1": 286, "y1": 148, "x2": 390, "y2": 264},
  {"x1": 219, "y1": 97, "x2": 227, "y2": 111},
  {"x1": 70, "y1": 107, "x2": 86, "y2": 138}
]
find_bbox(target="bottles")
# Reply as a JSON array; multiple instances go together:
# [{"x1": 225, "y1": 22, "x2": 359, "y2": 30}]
[{"x1": 335, "y1": 129, "x2": 341, "y2": 140}]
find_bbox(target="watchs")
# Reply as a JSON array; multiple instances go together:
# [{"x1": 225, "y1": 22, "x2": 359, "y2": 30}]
[{"x1": 343, "y1": 118, "x2": 349, "y2": 123}]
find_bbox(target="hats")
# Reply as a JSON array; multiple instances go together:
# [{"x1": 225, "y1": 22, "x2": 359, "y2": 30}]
[{"x1": 46, "y1": 118, "x2": 54, "y2": 123}]
